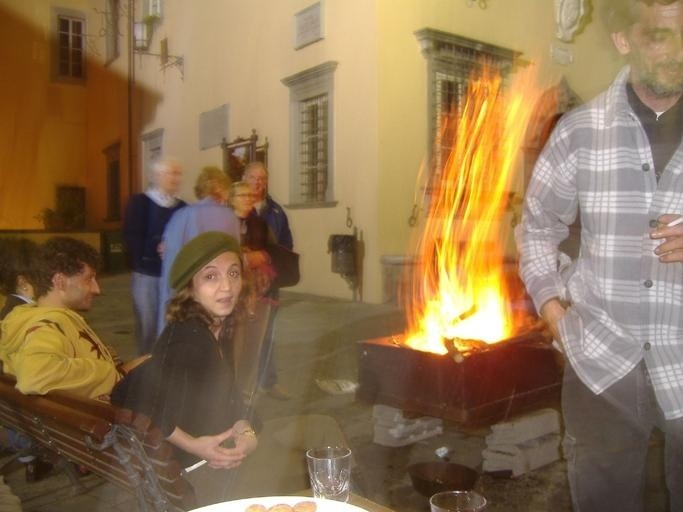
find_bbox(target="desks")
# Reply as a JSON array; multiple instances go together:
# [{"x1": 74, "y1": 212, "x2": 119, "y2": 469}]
[{"x1": 274, "y1": 480, "x2": 400, "y2": 512}]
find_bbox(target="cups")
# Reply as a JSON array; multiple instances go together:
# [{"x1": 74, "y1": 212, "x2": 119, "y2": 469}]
[
  {"x1": 306, "y1": 445, "x2": 351, "y2": 506},
  {"x1": 428, "y1": 491, "x2": 489, "y2": 512}
]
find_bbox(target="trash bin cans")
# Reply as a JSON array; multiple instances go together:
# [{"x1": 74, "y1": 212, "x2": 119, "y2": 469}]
[{"x1": 327, "y1": 234, "x2": 356, "y2": 274}]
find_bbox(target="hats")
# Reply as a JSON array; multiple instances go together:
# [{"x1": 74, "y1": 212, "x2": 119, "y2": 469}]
[{"x1": 166, "y1": 231, "x2": 241, "y2": 294}]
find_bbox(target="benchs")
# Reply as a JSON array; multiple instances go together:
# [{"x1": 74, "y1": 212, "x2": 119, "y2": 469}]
[{"x1": 0, "y1": 360, "x2": 228, "y2": 511}]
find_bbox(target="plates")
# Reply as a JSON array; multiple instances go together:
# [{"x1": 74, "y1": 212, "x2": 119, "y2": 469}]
[{"x1": 181, "y1": 494, "x2": 374, "y2": 512}]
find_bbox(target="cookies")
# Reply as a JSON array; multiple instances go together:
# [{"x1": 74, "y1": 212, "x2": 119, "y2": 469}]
[
  {"x1": 292, "y1": 501, "x2": 317, "y2": 512},
  {"x1": 267, "y1": 504, "x2": 292, "y2": 512},
  {"x1": 244, "y1": 500, "x2": 266, "y2": 512}
]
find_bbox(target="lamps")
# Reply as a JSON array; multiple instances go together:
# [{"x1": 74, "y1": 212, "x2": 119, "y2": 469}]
[{"x1": 132, "y1": 20, "x2": 152, "y2": 50}]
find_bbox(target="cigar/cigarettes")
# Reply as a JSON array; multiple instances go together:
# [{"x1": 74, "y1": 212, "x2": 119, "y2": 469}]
[{"x1": 667, "y1": 217, "x2": 683, "y2": 228}]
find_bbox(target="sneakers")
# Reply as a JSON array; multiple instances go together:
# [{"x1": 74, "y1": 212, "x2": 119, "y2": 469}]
[
  {"x1": 265, "y1": 384, "x2": 290, "y2": 399},
  {"x1": 25, "y1": 458, "x2": 53, "y2": 479}
]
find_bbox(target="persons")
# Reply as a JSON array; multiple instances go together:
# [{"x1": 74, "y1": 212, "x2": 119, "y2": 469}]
[{"x1": 514, "y1": 0, "x2": 683, "y2": 512}]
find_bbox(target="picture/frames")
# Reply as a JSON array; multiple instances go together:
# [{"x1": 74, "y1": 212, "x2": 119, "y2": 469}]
[
  {"x1": 252, "y1": 133, "x2": 270, "y2": 175},
  {"x1": 220, "y1": 125, "x2": 257, "y2": 187}
]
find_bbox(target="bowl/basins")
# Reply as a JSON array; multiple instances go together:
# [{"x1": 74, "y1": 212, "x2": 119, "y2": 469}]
[{"x1": 408, "y1": 461, "x2": 479, "y2": 497}]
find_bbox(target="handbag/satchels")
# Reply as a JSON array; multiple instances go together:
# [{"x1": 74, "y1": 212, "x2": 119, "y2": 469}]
[{"x1": 266, "y1": 244, "x2": 299, "y2": 287}]
